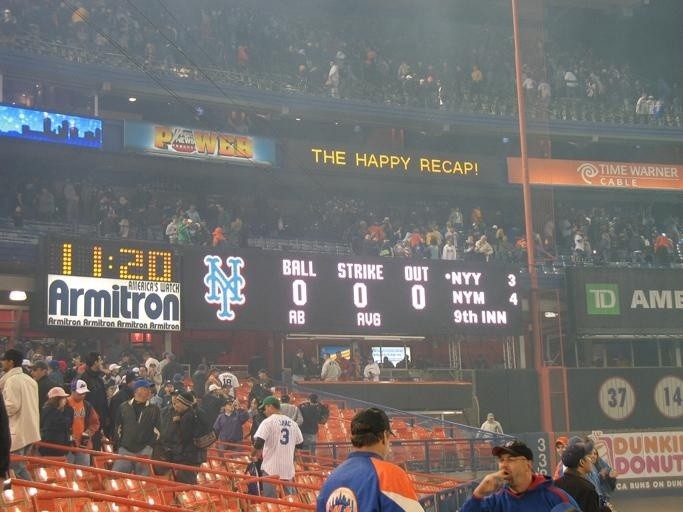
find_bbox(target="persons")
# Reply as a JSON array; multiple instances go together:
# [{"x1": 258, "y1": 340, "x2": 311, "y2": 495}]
[
  {"x1": 458, "y1": 406, "x2": 618, "y2": 510},
  {"x1": 0, "y1": 323, "x2": 456, "y2": 512},
  {"x1": 0, "y1": 0, "x2": 682, "y2": 258}
]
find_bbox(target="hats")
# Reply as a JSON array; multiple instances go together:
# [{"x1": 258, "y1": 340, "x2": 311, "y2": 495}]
[
  {"x1": 491, "y1": 441, "x2": 532, "y2": 461},
  {"x1": 555, "y1": 436, "x2": 568, "y2": 447},
  {"x1": 562, "y1": 441, "x2": 593, "y2": 468},
  {"x1": 350, "y1": 408, "x2": 397, "y2": 438},
  {"x1": 0, "y1": 349, "x2": 280, "y2": 410}
]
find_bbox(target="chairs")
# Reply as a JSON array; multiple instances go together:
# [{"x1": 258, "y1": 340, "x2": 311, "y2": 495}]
[{"x1": 0, "y1": 380, "x2": 494, "y2": 512}]
